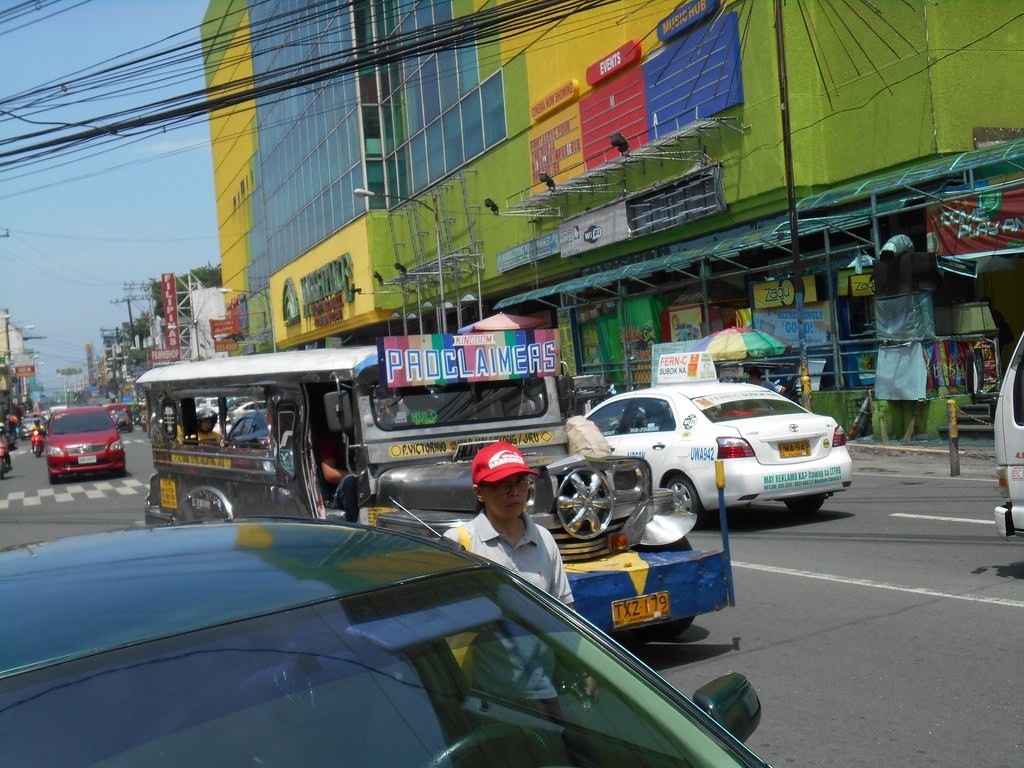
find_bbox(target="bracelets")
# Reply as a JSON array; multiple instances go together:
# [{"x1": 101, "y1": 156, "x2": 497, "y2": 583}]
[{"x1": 584, "y1": 674, "x2": 590, "y2": 679}]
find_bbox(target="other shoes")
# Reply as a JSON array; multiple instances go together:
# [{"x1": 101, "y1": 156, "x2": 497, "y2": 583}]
[{"x1": 5, "y1": 462, "x2": 10, "y2": 472}]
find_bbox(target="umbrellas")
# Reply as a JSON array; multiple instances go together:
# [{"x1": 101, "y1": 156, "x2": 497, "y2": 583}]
[
  {"x1": 690, "y1": 326, "x2": 793, "y2": 362},
  {"x1": 458, "y1": 312, "x2": 548, "y2": 335}
]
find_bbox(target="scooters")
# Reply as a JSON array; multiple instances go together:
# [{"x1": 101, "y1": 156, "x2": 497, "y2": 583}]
[
  {"x1": 28, "y1": 428, "x2": 45, "y2": 458},
  {"x1": 0, "y1": 441, "x2": 12, "y2": 480}
]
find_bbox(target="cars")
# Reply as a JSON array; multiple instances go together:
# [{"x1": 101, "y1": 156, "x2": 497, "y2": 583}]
[
  {"x1": 225, "y1": 411, "x2": 272, "y2": 450},
  {"x1": 994, "y1": 331, "x2": 1024, "y2": 539},
  {"x1": 583, "y1": 351, "x2": 852, "y2": 530},
  {"x1": 2, "y1": 522, "x2": 773, "y2": 768},
  {"x1": 227, "y1": 401, "x2": 267, "y2": 426},
  {"x1": 38, "y1": 406, "x2": 126, "y2": 484},
  {"x1": 110, "y1": 411, "x2": 133, "y2": 433},
  {"x1": 21, "y1": 418, "x2": 35, "y2": 440}
]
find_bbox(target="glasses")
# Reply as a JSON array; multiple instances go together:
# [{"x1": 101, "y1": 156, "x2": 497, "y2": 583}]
[{"x1": 479, "y1": 479, "x2": 533, "y2": 496}]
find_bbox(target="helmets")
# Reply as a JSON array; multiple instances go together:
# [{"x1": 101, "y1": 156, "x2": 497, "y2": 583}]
[
  {"x1": 0, "y1": 422, "x2": 5, "y2": 433},
  {"x1": 195, "y1": 405, "x2": 218, "y2": 433},
  {"x1": 33, "y1": 418, "x2": 39, "y2": 422}
]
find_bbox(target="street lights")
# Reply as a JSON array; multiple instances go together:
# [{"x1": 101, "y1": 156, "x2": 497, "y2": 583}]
[
  {"x1": 354, "y1": 188, "x2": 447, "y2": 333},
  {"x1": 220, "y1": 289, "x2": 276, "y2": 353}
]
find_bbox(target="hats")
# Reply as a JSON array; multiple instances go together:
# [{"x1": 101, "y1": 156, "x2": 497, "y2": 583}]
[{"x1": 472, "y1": 441, "x2": 542, "y2": 485}]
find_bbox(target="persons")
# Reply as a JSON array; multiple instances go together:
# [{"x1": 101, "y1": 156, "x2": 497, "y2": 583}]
[
  {"x1": 747, "y1": 366, "x2": 777, "y2": 393},
  {"x1": 319, "y1": 439, "x2": 357, "y2": 508},
  {"x1": 442, "y1": 443, "x2": 601, "y2": 719},
  {"x1": 110, "y1": 410, "x2": 119, "y2": 428},
  {"x1": 197, "y1": 408, "x2": 222, "y2": 443},
  {"x1": 29, "y1": 418, "x2": 44, "y2": 453},
  {"x1": 0, "y1": 422, "x2": 11, "y2": 466}
]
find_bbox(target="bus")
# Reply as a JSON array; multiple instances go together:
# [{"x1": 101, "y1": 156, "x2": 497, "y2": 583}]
[{"x1": 133, "y1": 329, "x2": 736, "y2": 639}]
